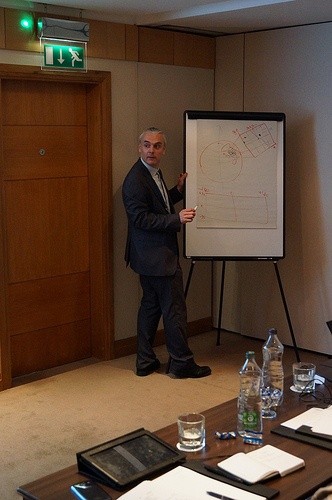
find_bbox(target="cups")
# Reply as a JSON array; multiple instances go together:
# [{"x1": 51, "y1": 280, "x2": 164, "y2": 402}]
[
  {"x1": 289, "y1": 362, "x2": 316, "y2": 390},
  {"x1": 177, "y1": 413, "x2": 206, "y2": 449}
]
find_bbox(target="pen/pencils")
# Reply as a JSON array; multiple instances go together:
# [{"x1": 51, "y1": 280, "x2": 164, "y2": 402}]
[{"x1": 207, "y1": 490, "x2": 235, "y2": 500}]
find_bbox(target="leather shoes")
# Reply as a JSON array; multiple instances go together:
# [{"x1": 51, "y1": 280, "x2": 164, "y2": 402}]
[
  {"x1": 137, "y1": 359, "x2": 160, "y2": 376},
  {"x1": 175, "y1": 362, "x2": 211, "y2": 378}
]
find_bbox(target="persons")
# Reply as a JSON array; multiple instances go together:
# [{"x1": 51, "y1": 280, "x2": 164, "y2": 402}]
[{"x1": 121, "y1": 127, "x2": 212, "y2": 379}]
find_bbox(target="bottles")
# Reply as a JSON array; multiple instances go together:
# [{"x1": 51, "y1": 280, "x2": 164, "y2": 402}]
[
  {"x1": 237, "y1": 352, "x2": 263, "y2": 439},
  {"x1": 262, "y1": 328, "x2": 283, "y2": 397}
]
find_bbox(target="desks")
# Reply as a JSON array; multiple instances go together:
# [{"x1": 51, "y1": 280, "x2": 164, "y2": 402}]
[{"x1": 17, "y1": 374, "x2": 332, "y2": 500}]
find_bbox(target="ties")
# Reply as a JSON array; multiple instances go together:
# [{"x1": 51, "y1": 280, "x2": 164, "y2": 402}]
[{"x1": 156, "y1": 172, "x2": 167, "y2": 204}]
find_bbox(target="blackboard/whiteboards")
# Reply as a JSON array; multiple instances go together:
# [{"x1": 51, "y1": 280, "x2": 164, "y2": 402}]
[{"x1": 181, "y1": 109, "x2": 288, "y2": 262}]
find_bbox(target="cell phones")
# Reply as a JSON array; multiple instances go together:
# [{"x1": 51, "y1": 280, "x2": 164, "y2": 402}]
[{"x1": 70, "y1": 479, "x2": 114, "y2": 500}]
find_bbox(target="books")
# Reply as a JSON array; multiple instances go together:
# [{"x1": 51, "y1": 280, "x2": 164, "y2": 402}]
[
  {"x1": 271, "y1": 405, "x2": 332, "y2": 450},
  {"x1": 217, "y1": 444, "x2": 306, "y2": 485},
  {"x1": 115, "y1": 460, "x2": 280, "y2": 500}
]
